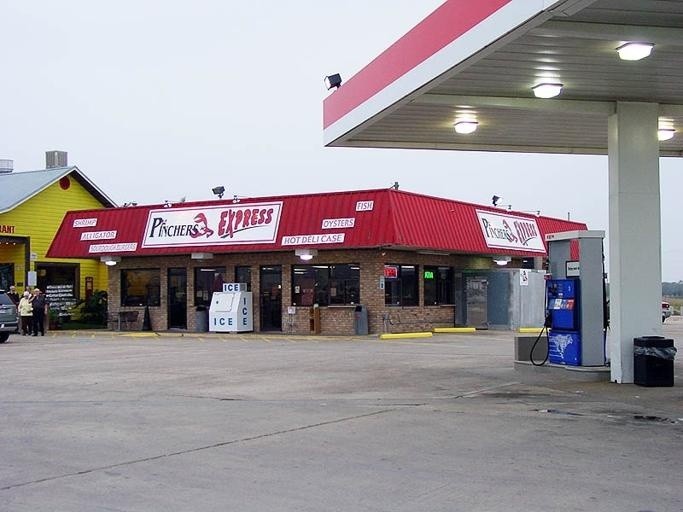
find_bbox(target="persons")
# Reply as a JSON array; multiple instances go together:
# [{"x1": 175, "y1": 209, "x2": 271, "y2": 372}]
[
  {"x1": 5, "y1": 286, "x2": 20, "y2": 335},
  {"x1": 20, "y1": 286, "x2": 33, "y2": 334},
  {"x1": 17, "y1": 291, "x2": 33, "y2": 335},
  {"x1": 27, "y1": 288, "x2": 46, "y2": 336}
]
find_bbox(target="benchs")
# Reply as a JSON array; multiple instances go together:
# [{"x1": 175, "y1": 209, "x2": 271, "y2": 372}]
[{"x1": 106, "y1": 311, "x2": 138, "y2": 330}]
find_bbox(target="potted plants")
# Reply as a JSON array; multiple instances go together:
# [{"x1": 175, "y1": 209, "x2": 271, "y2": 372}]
[{"x1": 48, "y1": 309, "x2": 57, "y2": 328}]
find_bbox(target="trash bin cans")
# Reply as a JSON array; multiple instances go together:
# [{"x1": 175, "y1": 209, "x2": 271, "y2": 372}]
[
  {"x1": 354, "y1": 304, "x2": 369, "y2": 335},
  {"x1": 634, "y1": 336, "x2": 674, "y2": 387},
  {"x1": 195, "y1": 305, "x2": 209, "y2": 333}
]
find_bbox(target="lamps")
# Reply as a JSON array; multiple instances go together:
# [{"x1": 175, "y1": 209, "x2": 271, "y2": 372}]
[
  {"x1": 211, "y1": 186, "x2": 226, "y2": 199},
  {"x1": 100, "y1": 256, "x2": 121, "y2": 266},
  {"x1": 322, "y1": 71, "x2": 341, "y2": 91},
  {"x1": 492, "y1": 195, "x2": 504, "y2": 206},
  {"x1": 295, "y1": 249, "x2": 319, "y2": 260}
]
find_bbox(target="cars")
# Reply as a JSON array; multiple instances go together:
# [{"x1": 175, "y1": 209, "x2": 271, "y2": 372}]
[
  {"x1": 0, "y1": 288, "x2": 18, "y2": 343},
  {"x1": 606, "y1": 300, "x2": 671, "y2": 323}
]
